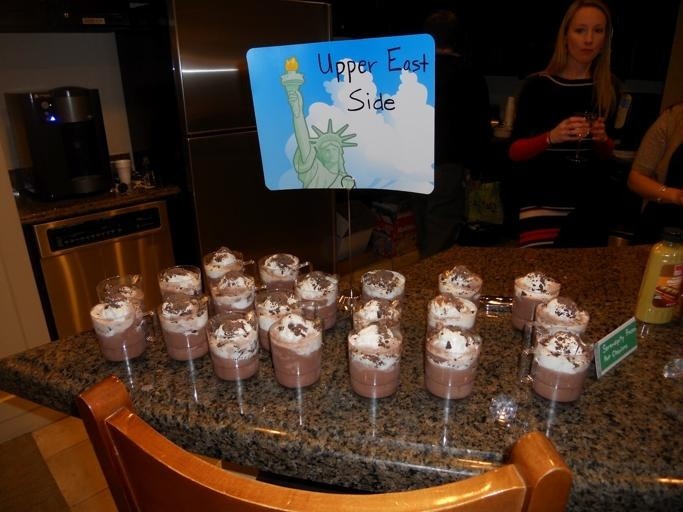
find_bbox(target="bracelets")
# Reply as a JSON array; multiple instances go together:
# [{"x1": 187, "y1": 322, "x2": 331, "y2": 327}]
[
  {"x1": 657, "y1": 184, "x2": 668, "y2": 203},
  {"x1": 548, "y1": 130, "x2": 552, "y2": 144}
]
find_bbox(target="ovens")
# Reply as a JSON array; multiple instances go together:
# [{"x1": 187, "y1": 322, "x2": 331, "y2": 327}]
[{"x1": 31, "y1": 200, "x2": 176, "y2": 340}]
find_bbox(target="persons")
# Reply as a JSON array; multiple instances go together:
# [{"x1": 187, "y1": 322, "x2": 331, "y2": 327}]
[
  {"x1": 411, "y1": 12, "x2": 495, "y2": 261},
  {"x1": 505, "y1": 0, "x2": 622, "y2": 251},
  {"x1": 626, "y1": 103, "x2": 683, "y2": 246}
]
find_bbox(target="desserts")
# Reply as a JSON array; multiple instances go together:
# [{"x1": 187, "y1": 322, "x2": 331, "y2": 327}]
[
  {"x1": 255, "y1": 253, "x2": 338, "y2": 387},
  {"x1": 157, "y1": 265, "x2": 208, "y2": 362},
  {"x1": 90, "y1": 275, "x2": 157, "y2": 363},
  {"x1": 203, "y1": 246, "x2": 268, "y2": 381},
  {"x1": 348, "y1": 269, "x2": 406, "y2": 398}
]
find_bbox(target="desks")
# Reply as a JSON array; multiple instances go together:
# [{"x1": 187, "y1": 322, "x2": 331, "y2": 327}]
[{"x1": 0, "y1": 245, "x2": 683, "y2": 510}]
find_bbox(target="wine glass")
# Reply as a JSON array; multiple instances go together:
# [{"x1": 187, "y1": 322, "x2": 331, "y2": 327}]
[{"x1": 567, "y1": 111, "x2": 598, "y2": 161}]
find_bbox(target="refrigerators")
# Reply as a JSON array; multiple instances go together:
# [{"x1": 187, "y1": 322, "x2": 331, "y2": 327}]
[{"x1": 108, "y1": 1, "x2": 338, "y2": 290}]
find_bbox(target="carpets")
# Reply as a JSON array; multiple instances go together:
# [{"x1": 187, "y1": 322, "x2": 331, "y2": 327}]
[{"x1": 0, "y1": 432, "x2": 71, "y2": 512}]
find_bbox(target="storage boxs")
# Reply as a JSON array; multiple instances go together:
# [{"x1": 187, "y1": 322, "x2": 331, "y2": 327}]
[{"x1": 335, "y1": 212, "x2": 371, "y2": 262}]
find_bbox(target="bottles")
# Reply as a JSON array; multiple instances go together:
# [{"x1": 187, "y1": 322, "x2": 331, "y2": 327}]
[
  {"x1": 501, "y1": 95, "x2": 515, "y2": 125},
  {"x1": 633, "y1": 225, "x2": 682, "y2": 325}
]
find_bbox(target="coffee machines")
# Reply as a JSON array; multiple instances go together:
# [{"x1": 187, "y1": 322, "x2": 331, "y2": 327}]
[{"x1": 4, "y1": 83, "x2": 114, "y2": 203}]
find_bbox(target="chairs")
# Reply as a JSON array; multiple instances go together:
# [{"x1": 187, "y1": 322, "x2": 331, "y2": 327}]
[{"x1": 78, "y1": 373, "x2": 573, "y2": 512}]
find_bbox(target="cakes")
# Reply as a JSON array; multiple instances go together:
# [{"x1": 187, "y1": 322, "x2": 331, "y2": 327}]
[
  {"x1": 510, "y1": 269, "x2": 562, "y2": 331},
  {"x1": 531, "y1": 287, "x2": 590, "y2": 349},
  {"x1": 438, "y1": 264, "x2": 483, "y2": 311},
  {"x1": 425, "y1": 294, "x2": 478, "y2": 334}
]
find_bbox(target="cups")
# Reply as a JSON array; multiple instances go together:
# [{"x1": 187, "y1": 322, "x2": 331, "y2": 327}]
[
  {"x1": 88, "y1": 301, "x2": 149, "y2": 364},
  {"x1": 95, "y1": 276, "x2": 144, "y2": 311},
  {"x1": 154, "y1": 249, "x2": 404, "y2": 399},
  {"x1": 116, "y1": 158, "x2": 131, "y2": 186},
  {"x1": 532, "y1": 301, "x2": 590, "y2": 350},
  {"x1": 510, "y1": 275, "x2": 563, "y2": 335},
  {"x1": 438, "y1": 273, "x2": 483, "y2": 308},
  {"x1": 425, "y1": 297, "x2": 478, "y2": 339}
]
